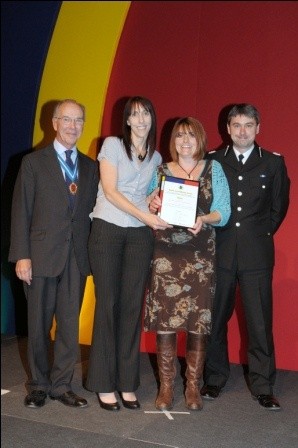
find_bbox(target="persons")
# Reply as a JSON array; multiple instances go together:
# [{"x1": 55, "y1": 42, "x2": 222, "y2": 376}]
[
  {"x1": 7, "y1": 99, "x2": 101, "y2": 409},
  {"x1": 200, "y1": 103, "x2": 291, "y2": 411},
  {"x1": 84, "y1": 96, "x2": 174, "y2": 411},
  {"x1": 149, "y1": 116, "x2": 231, "y2": 412}
]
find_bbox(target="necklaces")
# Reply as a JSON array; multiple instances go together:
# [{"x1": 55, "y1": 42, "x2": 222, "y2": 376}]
[
  {"x1": 176, "y1": 159, "x2": 200, "y2": 179},
  {"x1": 132, "y1": 141, "x2": 146, "y2": 160}
]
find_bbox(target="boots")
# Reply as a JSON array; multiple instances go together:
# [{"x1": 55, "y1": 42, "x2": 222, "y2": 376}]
[
  {"x1": 154, "y1": 333, "x2": 178, "y2": 412},
  {"x1": 183, "y1": 332, "x2": 207, "y2": 411}
]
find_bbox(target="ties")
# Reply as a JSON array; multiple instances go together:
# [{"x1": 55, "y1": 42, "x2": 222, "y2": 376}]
[
  {"x1": 64, "y1": 150, "x2": 78, "y2": 207},
  {"x1": 237, "y1": 154, "x2": 244, "y2": 168}
]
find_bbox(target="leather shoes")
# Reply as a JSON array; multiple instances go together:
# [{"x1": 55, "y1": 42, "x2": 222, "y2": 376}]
[
  {"x1": 49, "y1": 389, "x2": 88, "y2": 409},
  {"x1": 201, "y1": 383, "x2": 220, "y2": 400},
  {"x1": 23, "y1": 390, "x2": 47, "y2": 409},
  {"x1": 253, "y1": 393, "x2": 281, "y2": 410},
  {"x1": 96, "y1": 391, "x2": 119, "y2": 411},
  {"x1": 118, "y1": 390, "x2": 141, "y2": 409}
]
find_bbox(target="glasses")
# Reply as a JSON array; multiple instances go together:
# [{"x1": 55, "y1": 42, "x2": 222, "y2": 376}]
[{"x1": 54, "y1": 116, "x2": 85, "y2": 126}]
